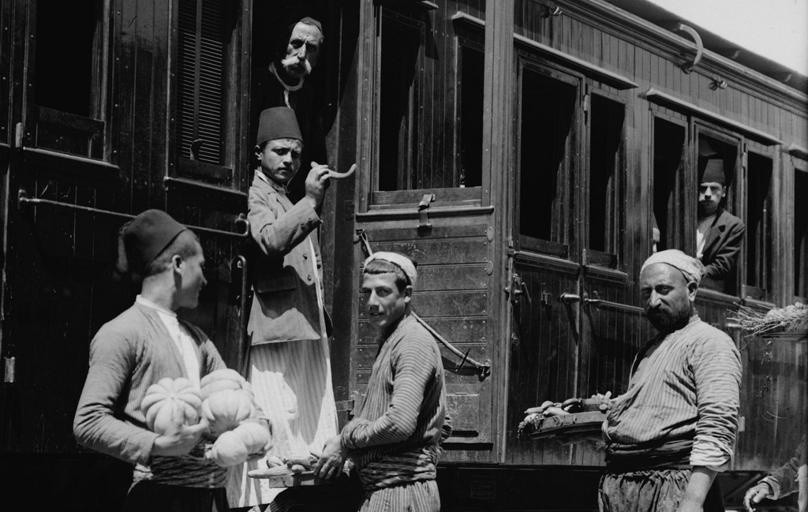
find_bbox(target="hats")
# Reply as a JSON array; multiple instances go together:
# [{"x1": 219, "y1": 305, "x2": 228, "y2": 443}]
[
  {"x1": 698, "y1": 159, "x2": 725, "y2": 185},
  {"x1": 639, "y1": 249, "x2": 707, "y2": 288},
  {"x1": 363, "y1": 251, "x2": 417, "y2": 291},
  {"x1": 256, "y1": 107, "x2": 304, "y2": 145},
  {"x1": 121, "y1": 209, "x2": 187, "y2": 275}
]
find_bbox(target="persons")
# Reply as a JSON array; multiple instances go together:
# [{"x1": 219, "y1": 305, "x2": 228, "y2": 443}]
[
  {"x1": 250, "y1": 15, "x2": 339, "y2": 204},
  {"x1": 696, "y1": 158, "x2": 747, "y2": 293},
  {"x1": 743, "y1": 437, "x2": 808, "y2": 512},
  {"x1": 225, "y1": 106, "x2": 339, "y2": 509},
  {"x1": 71, "y1": 208, "x2": 274, "y2": 512},
  {"x1": 596, "y1": 249, "x2": 743, "y2": 512},
  {"x1": 313, "y1": 251, "x2": 453, "y2": 512}
]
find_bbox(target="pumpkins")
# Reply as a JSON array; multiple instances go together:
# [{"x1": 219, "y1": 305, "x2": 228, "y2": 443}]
[{"x1": 140, "y1": 369, "x2": 270, "y2": 466}]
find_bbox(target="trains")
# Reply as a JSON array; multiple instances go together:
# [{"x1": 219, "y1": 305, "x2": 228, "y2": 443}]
[{"x1": 0, "y1": 3, "x2": 808, "y2": 511}]
